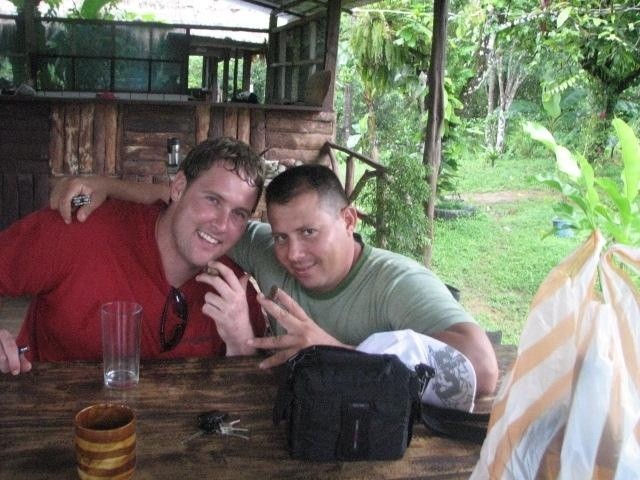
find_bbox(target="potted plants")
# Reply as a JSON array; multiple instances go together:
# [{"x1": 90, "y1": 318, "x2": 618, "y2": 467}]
[{"x1": 531, "y1": 173, "x2": 581, "y2": 238}]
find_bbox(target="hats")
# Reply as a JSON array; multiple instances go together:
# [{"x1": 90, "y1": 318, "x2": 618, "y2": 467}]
[{"x1": 356, "y1": 327, "x2": 476, "y2": 413}]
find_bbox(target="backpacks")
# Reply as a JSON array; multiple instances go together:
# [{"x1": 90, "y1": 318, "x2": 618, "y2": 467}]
[{"x1": 272, "y1": 345, "x2": 423, "y2": 462}]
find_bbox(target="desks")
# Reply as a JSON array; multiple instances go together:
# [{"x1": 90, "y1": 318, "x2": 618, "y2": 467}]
[
  {"x1": 0, "y1": 337, "x2": 518, "y2": 480},
  {"x1": 167, "y1": 173, "x2": 274, "y2": 224}
]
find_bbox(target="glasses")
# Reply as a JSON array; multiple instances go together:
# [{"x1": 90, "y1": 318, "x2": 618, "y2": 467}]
[{"x1": 160, "y1": 286, "x2": 189, "y2": 352}]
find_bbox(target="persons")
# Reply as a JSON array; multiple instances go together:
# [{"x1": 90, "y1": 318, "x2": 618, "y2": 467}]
[
  {"x1": 0, "y1": 133, "x2": 268, "y2": 377},
  {"x1": 47, "y1": 162, "x2": 499, "y2": 397}
]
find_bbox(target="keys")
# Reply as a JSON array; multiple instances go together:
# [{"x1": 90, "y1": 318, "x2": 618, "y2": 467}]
[{"x1": 179, "y1": 410, "x2": 250, "y2": 449}]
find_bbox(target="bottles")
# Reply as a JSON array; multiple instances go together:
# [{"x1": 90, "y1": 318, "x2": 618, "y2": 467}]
[{"x1": 167, "y1": 137, "x2": 180, "y2": 167}]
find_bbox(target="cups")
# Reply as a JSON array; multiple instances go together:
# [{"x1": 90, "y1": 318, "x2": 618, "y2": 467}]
[
  {"x1": 74, "y1": 401, "x2": 138, "y2": 480},
  {"x1": 100, "y1": 301, "x2": 144, "y2": 391}
]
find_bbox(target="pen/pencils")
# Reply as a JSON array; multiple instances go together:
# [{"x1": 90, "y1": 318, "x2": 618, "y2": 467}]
[{"x1": 19, "y1": 344, "x2": 30, "y2": 353}]
